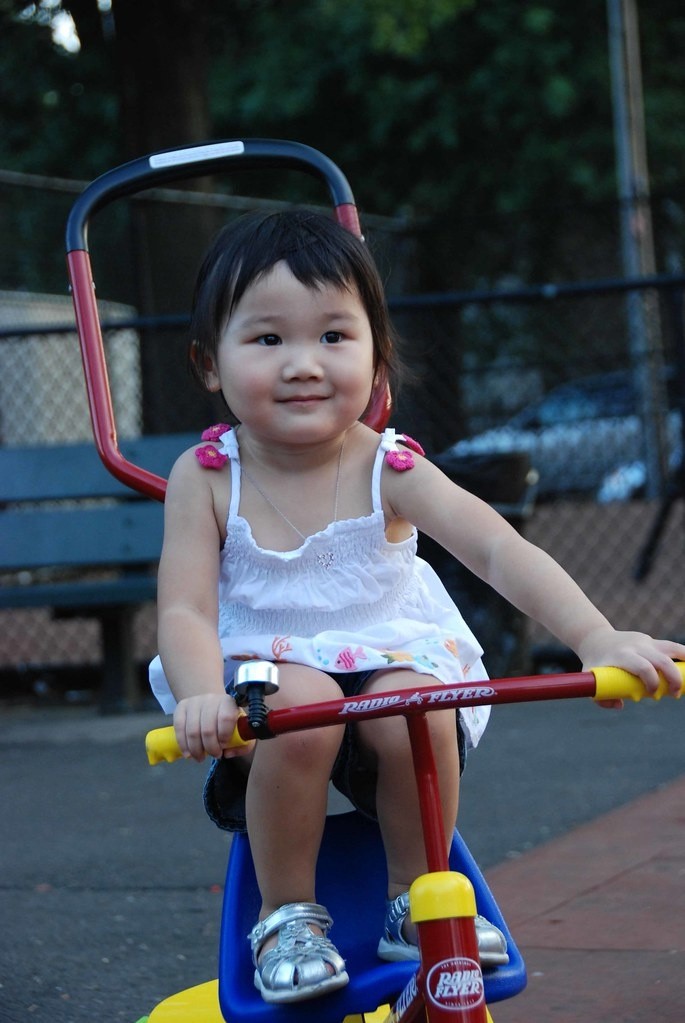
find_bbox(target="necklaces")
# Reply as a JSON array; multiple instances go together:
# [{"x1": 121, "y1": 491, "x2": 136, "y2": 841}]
[{"x1": 232, "y1": 423, "x2": 345, "y2": 569}]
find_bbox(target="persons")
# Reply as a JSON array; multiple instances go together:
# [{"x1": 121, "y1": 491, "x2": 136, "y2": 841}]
[{"x1": 149, "y1": 208, "x2": 685, "y2": 1004}]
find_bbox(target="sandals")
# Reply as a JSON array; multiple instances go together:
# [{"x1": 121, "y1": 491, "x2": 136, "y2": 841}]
[
  {"x1": 376, "y1": 891, "x2": 509, "y2": 966},
  {"x1": 247, "y1": 901, "x2": 350, "y2": 1003}
]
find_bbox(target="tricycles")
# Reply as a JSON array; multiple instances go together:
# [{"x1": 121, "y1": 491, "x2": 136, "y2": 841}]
[{"x1": 66, "y1": 137, "x2": 685, "y2": 1022}]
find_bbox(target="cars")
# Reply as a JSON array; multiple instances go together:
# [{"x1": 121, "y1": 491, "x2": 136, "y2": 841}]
[{"x1": 431, "y1": 362, "x2": 685, "y2": 503}]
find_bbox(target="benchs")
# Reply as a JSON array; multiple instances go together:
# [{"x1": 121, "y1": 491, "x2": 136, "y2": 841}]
[{"x1": 0, "y1": 431, "x2": 212, "y2": 719}]
